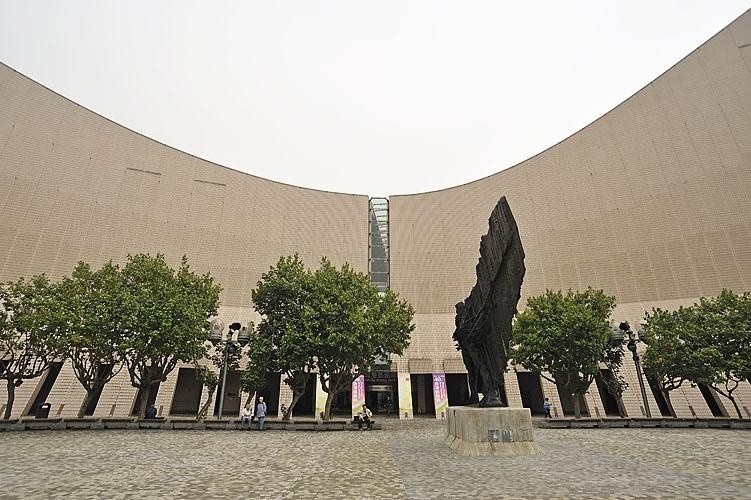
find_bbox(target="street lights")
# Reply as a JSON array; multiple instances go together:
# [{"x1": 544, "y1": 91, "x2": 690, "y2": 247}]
[
  {"x1": 206, "y1": 317, "x2": 253, "y2": 419},
  {"x1": 611, "y1": 317, "x2": 657, "y2": 418}
]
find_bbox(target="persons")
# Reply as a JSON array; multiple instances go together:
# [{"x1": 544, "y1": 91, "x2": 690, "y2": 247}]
[
  {"x1": 358, "y1": 404, "x2": 373, "y2": 431},
  {"x1": 256, "y1": 396, "x2": 268, "y2": 430},
  {"x1": 145, "y1": 405, "x2": 157, "y2": 420},
  {"x1": 543, "y1": 398, "x2": 553, "y2": 418},
  {"x1": 383, "y1": 396, "x2": 392, "y2": 418},
  {"x1": 240, "y1": 404, "x2": 255, "y2": 431}
]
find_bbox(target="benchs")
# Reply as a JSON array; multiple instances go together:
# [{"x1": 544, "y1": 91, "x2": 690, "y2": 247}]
[{"x1": 537, "y1": 413, "x2": 751, "y2": 431}]
